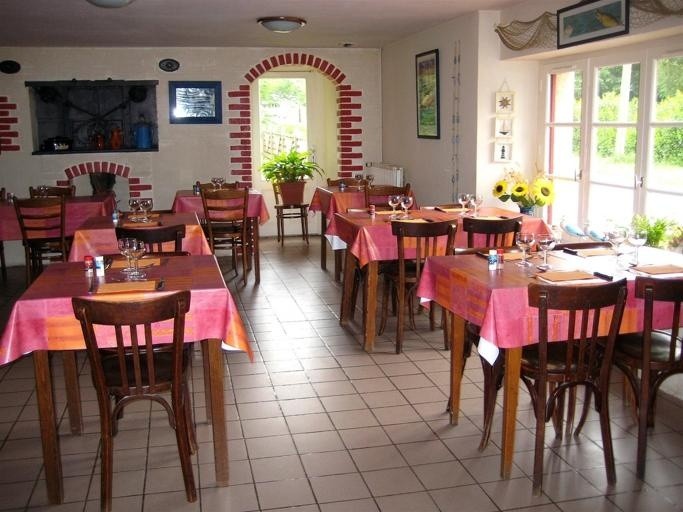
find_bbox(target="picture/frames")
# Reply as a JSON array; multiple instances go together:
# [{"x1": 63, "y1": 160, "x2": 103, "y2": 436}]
[
  {"x1": 169, "y1": 80, "x2": 223, "y2": 125},
  {"x1": 495, "y1": 117, "x2": 514, "y2": 137},
  {"x1": 557, "y1": 0, "x2": 629, "y2": 49},
  {"x1": 492, "y1": 143, "x2": 513, "y2": 162},
  {"x1": 496, "y1": 92, "x2": 516, "y2": 115},
  {"x1": 414, "y1": 49, "x2": 440, "y2": 139}
]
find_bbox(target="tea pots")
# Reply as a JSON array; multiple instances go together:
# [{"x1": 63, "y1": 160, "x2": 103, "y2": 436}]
[{"x1": 131, "y1": 119, "x2": 154, "y2": 150}]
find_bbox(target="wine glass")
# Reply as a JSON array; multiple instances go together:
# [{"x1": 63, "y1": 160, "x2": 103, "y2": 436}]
[
  {"x1": 514, "y1": 232, "x2": 553, "y2": 270},
  {"x1": 211, "y1": 176, "x2": 224, "y2": 192},
  {"x1": 40, "y1": 186, "x2": 49, "y2": 199},
  {"x1": 627, "y1": 228, "x2": 648, "y2": 267},
  {"x1": 117, "y1": 237, "x2": 146, "y2": 279},
  {"x1": 366, "y1": 174, "x2": 374, "y2": 189},
  {"x1": 609, "y1": 228, "x2": 627, "y2": 269},
  {"x1": 387, "y1": 195, "x2": 413, "y2": 220},
  {"x1": 354, "y1": 173, "x2": 363, "y2": 190},
  {"x1": 458, "y1": 191, "x2": 483, "y2": 218},
  {"x1": 128, "y1": 198, "x2": 153, "y2": 222}
]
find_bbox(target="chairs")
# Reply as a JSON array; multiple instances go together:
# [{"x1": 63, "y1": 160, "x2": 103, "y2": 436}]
[{"x1": 273, "y1": 182, "x2": 309, "y2": 246}]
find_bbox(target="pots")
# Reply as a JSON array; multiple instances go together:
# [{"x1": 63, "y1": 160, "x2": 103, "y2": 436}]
[{"x1": 43, "y1": 136, "x2": 73, "y2": 151}]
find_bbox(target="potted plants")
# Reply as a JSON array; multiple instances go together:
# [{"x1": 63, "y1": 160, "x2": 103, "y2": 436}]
[{"x1": 260, "y1": 151, "x2": 324, "y2": 205}]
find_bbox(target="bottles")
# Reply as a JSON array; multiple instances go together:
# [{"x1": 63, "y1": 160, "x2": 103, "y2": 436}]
[
  {"x1": 7, "y1": 193, "x2": 14, "y2": 205},
  {"x1": 369, "y1": 204, "x2": 376, "y2": 219},
  {"x1": 83, "y1": 256, "x2": 105, "y2": 277},
  {"x1": 488, "y1": 249, "x2": 504, "y2": 271},
  {"x1": 193, "y1": 180, "x2": 201, "y2": 196},
  {"x1": 112, "y1": 209, "x2": 118, "y2": 223},
  {"x1": 339, "y1": 180, "x2": 345, "y2": 192}
]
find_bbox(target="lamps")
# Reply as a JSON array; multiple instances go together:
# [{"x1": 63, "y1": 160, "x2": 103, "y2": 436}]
[
  {"x1": 257, "y1": 16, "x2": 307, "y2": 33},
  {"x1": 87, "y1": 0, "x2": 133, "y2": 8}
]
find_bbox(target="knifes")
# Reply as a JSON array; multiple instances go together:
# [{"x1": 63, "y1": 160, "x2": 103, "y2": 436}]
[
  {"x1": 563, "y1": 247, "x2": 586, "y2": 258},
  {"x1": 105, "y1": 259, "x2": 111, "y2": 270},
  {"x1": 156, "y1": 275, "x2": 166, "y2": 291}
]
